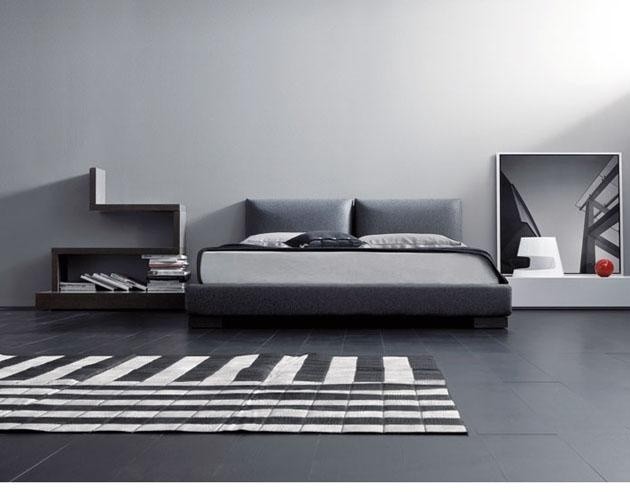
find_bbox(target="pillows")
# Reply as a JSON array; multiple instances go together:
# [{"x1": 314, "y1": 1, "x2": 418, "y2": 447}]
[
  {"x1": 284, "y1": 230, "x2": 366, "y2": 248},
  {"x1": 357, "y1": 233, "x2": 466, "y2": 250},
  {"x1": 354, "y1": 198, "x2": 462, "y2": 241},
  {"x1": 238, "y1": 232, "x2": 308, "y2": 249},
  {"x1": 245, "y1": 199, "x2": 353, "y2": 240}
]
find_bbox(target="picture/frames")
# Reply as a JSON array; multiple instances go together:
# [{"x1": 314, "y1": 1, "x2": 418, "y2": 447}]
[{"x1": 496, "y1": 152, "x2": 624, "y2": 277}]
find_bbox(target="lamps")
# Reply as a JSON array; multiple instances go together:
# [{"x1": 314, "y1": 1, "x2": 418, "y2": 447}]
[{"x1": 513, "y1": 236, "x2": 563, "y2": 277}]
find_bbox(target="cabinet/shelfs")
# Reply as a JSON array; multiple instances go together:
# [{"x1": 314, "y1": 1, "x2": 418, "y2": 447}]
[
  {"x1": 504, "y1": 276, "x2": 630, "y2": 310},
  {"x1": 35, "y1": 167, "x2": 192, "y2": 311}
]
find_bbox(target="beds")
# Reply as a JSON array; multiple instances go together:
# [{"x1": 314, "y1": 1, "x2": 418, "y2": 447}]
[{"x1": 183, "y1": 197, "x2": 511, "y2": 330}]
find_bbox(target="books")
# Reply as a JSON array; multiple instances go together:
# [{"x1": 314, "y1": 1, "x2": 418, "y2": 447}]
[{"x1": 58, "y1": 255, "x2": 193, "y2": 295}]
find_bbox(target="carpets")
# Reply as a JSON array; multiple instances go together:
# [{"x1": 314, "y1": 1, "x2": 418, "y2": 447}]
[{"x1": 0, "y1": 351, "x2": 467, "y2": 436}]
